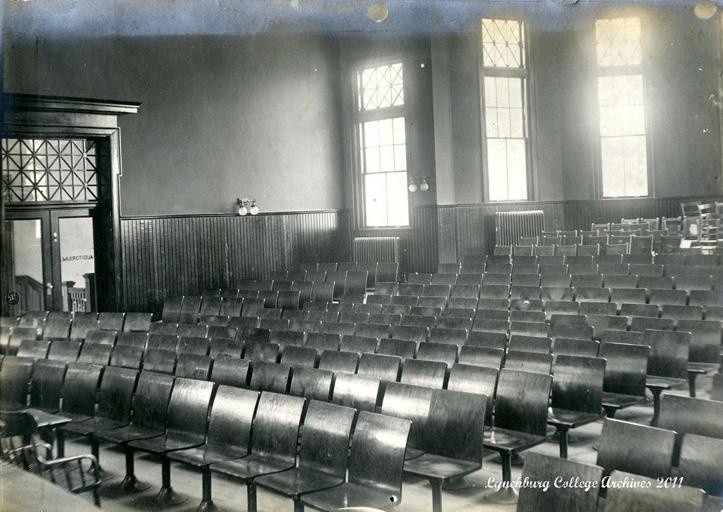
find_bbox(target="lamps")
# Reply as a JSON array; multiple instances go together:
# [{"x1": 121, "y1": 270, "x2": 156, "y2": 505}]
[
  {"x1": 237, "y1": 198, "x2": 258, "y2": 216},
  {"x1": 408, "y1": 175, "x2": 431, "y2": 193}
]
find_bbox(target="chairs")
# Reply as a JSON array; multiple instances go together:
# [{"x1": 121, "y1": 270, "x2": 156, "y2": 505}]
[{"x1": 0, "y1": 200, "x2": 722, "y2": 512}]
